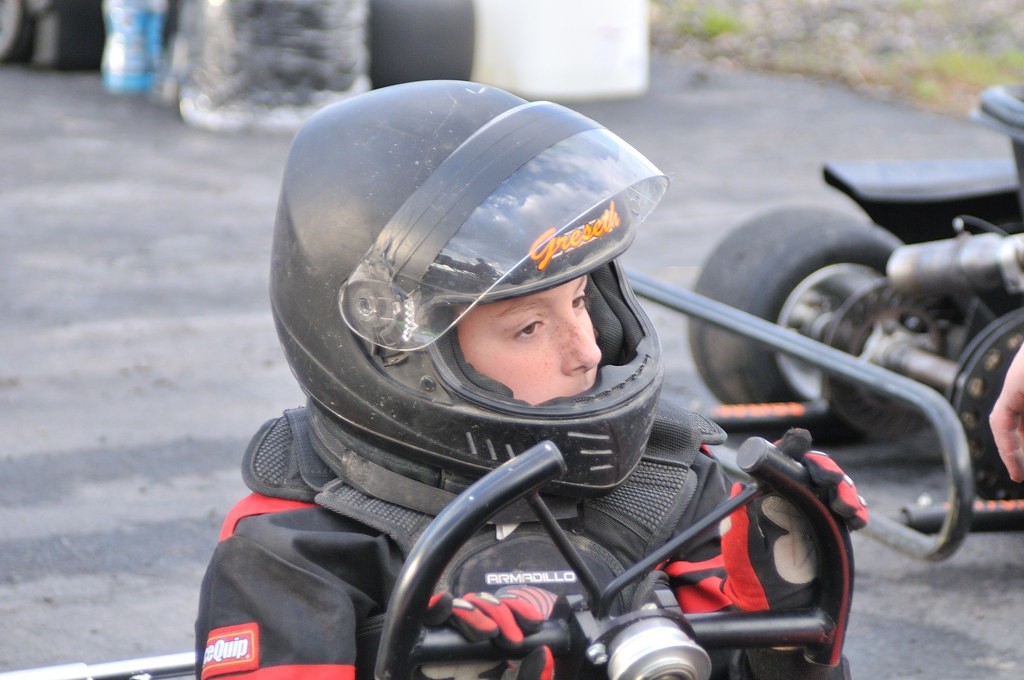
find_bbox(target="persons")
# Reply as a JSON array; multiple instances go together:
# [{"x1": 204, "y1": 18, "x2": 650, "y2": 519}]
[{"x1": 195, "y1": 80, "x2": 868, "y2": 680}]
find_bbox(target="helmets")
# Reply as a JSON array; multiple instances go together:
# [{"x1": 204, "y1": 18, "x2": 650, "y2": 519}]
[{"x1": 270, "y1": 80, "x2": 669, "y2": 499}]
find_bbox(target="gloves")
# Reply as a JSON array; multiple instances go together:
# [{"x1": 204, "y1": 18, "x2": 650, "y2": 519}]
[
  {"x1": 409, "y1": 585, "x2": 588, "y2": 680},
  {"x1": 720, "y1": 428, "x2": 868, "y2": 647}
]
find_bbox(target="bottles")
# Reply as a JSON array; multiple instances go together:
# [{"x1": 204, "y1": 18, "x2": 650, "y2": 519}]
[{"x1": 100, "y1": 0, "x2": 167, "y2": 94}]
